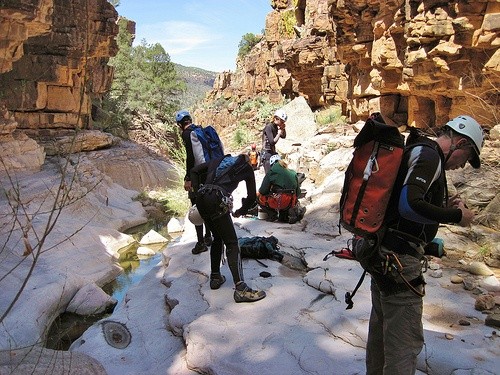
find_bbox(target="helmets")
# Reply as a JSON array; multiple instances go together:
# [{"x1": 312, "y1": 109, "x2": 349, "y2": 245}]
[
  {"x1": 273, "y1": 110, "x2": 288, "y2": 122},
  {"x1": 445, "y1": 115, "x2": 485, "y2": 169},
  {"x1": 176, "y1": 110, "x2": 191, "y2": 123},
  {"x1": 188, "y1": 204, "x2": 205, "y2": 226},
  {"x1": 252, "y1": 144, "x2": 256, "y2": 149},
  {"x1": 269, "y1": 155, "x2": 282, "y2": 166}
]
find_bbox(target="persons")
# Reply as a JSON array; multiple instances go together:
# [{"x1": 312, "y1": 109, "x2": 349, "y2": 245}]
[
  {"x1": 256, "y1": 155, "x2": 300, "y2": 223},
  {"x1": 190, "y1": 153, "x2": 266, "y2": 303},
  {"x1": 260, "y1": 110, "x2": 288, "y2": 173},
  {"x1": 176, "y1": 110, "x2": 213, "y2": 254},
  {"x1": 365, "y1": 115, "x2": 485, "y2": 375},
  {"x1": 248, "y1": 144, "x2": 261, "y2": 170}
]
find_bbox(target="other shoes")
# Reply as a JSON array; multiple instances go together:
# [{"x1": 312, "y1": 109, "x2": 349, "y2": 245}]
[
  {"x1": 210, "y1": 275, "x2": 226, "y2": 289},
  {"x1": 234, "y1": 287, "x2": 265, "y2": 303},
  {"x1": 203, "y1": 235, "x2": 212, "y2": 246},
  {"x1": 192, "y1": 242, "x2": 208, "y2": 254}
]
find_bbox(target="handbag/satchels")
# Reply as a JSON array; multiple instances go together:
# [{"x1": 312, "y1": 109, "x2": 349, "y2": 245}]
[
  {"x1": 426, "y1": 237, "x2": 445, "y2": 258},
  {"x1": 268, "y1": 189, "x2": 294, "y2": 213}
]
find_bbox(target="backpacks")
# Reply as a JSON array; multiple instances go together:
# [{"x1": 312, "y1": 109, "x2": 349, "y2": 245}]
[
  {"x1": 250, "y1": 152, "x2": 258, "y2": 164},
  {"x1": 204, "y1": 126, "x2": 224, "y2": 163},
  {"x1": 338, "y1": 118, "x2": 449, "y2": 236},
  {"x1": 239, "y1": 236, "x2": 284, "y2": 263}
]
